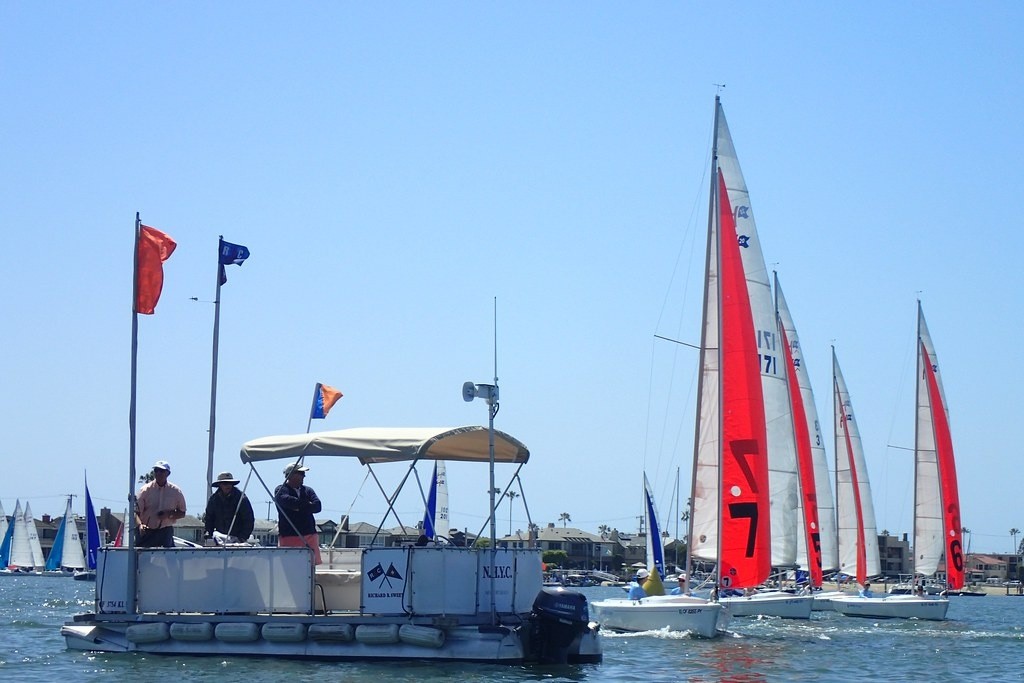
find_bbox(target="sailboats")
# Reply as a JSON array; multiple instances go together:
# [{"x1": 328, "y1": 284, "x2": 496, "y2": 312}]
[
  {"x1": 623, "y1": 470, "x2": 665, "y2": 593},
  {"x1": 760, "y1": 269, "x2": 836, "y2": 612},
  {"x1": 685, "y1": 95, "x2": 820, "y2": 619},
  {"x1": 803, "y1": 345, "x2": 882, "y2": 609},
  {"x1": 0, "y1": 467, "x2": 129, "y2": 579},
  {"x1": 589, "y1": 161, "x2": 774, "y2": 638},
  {"x1": 825, "y1": 288, "x2": 965, "y2": 620},
  {"x1": 652, "y1": 467, "x2": 715, "y2": 589}
]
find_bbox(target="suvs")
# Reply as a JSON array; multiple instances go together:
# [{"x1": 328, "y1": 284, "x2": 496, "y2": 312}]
[{"x1": 1003, "y1": 580, "x2": 1022, "y2": 587}]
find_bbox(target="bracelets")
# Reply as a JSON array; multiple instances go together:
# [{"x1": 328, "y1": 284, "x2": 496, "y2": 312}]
[
  {"x1": 138, "y1": 523, "x2": 143, "y2": 531},
  {"x1": 134, "y1": 462, "x2": 187, "y2": 614}
]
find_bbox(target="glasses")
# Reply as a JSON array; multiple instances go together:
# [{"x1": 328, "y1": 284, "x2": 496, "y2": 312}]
[
  {"x1": 154, "y1": 468, "x2": 166, "y2": 473},
  {"x1": 679, "y1": 579, "x2": 685, "y2": 582}
]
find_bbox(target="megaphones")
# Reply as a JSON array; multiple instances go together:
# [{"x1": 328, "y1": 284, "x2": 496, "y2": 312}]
[{"x1": 462, "y1": 382, "x2": 478, "y2": 402}]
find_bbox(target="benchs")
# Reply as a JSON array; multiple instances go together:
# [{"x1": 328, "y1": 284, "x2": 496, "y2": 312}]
[{"x1": 314, "y1": 573, "x2": 361, "y2": 613}]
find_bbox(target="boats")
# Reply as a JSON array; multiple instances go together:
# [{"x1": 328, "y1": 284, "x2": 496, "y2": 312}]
[
  {"x1": 887, "y1": 571, "x2": 988, "y2": 596},
  {"x1": 543, "y1": 571, "x2": 562, "y2": 585},
  {"x1": 561, "y1": 573, "x2": 599, "y2": 587},
  {"x1": 59, "y1": 214, "x2": 606, "y2": 662}
]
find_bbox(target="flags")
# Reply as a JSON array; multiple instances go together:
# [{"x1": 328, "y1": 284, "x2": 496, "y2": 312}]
[
  {"x1": 312, "y1": 384, "x2": 344, "y2": 418},
  {"x1": 136, "y1": 224, "x2": 177, "y2": 315},
  {"x1": 221, "y1": 240, "x2": 251, "y2": 286}
]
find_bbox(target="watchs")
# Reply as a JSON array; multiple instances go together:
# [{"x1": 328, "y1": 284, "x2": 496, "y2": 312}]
[{"x1": 169, "y1": 511, "x2": 173, "y2": 519}]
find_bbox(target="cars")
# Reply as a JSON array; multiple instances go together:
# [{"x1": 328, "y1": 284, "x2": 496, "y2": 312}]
[{"x1": 831, "y1": 571, "x2": 893, "y2": 583}]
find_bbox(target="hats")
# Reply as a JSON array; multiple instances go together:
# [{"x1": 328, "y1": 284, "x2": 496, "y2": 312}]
[
  {"x1": 864, "y1": 581, "x2": 870, "y2": 585},
  {"x1": 636, "y1": 569, "x2": 651, "y2": 578},
  {"x1": 284, "y1": 462, "x2": 310, "y2": 478},
  {"x1": 211, "y1": 472, "x2": 240, "y2": 487},
  {"x1": 678, "y1": 574, "x2": 686, "y2": 580},
  {"x1": 152, "y1": 461, "x2": 170, "y2": 473}
]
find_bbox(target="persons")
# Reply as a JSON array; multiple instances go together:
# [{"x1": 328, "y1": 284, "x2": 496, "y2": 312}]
[
  {"x1": 798, "y1": 582, "x2": 811, "y2": 595},
  {"x1": 628, "y1": 569, "x2": 649, "y2": 599},
  {"x1": 671, "y1": 574, "x2": 696, "y2": 596},
  {"x1": 275, "y1": 463, "x2": 321, "y2": 613},
  {"x1": 916, "y1": 586, "x2": 926, "y2": 597},
  {"x1": 859, "y1": 581, "x2": 872, "y2": 598},
  {"x1": 206, "y1": 473, "x2": 254, "y2": 545},
  {"x1": 721, "y1": 587, "x2": 758, "y2": 598},
  {"x1": 770, "y1": 578, "x2": 779, "y2": 588}
]
